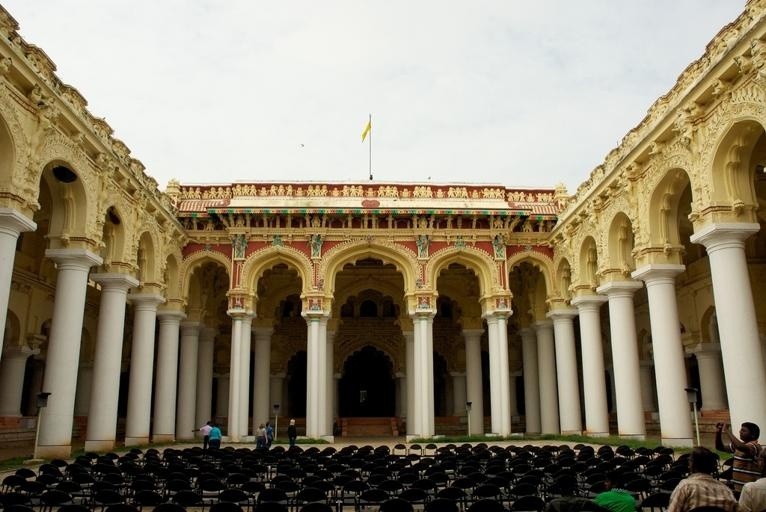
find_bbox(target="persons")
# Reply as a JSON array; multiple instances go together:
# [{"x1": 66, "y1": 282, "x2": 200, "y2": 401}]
[
  {"x1": 191, "y1": 421, "x2": 212, "y2": 449},
  {"x1": 208, "y1": 423, "x2": 223, "y2": 448},
  {"x1": 593, "y1": 472, "x2": 637, "y2": 512},
  {"x1": 715, "y1": 420, "x2": 763, "y2": 500},
  {"x1": 264, "y1": 421, "x2": 273, "y2": 447},
  {"x1": 737, "y1": 446, "x2": 766, "y2": 512},
  {"x1": 255, "y1": 423, "x2": 266, "y2": 448},
  {"x1": 665, "y1": 446, "x2": 738, "y2": 512},
  {"x1": 287, "y1": 418, "x2": 297, "y2": 446}
]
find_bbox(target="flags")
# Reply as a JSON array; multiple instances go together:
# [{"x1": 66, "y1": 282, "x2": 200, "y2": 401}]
[{"x1": 361, "y1": 119, "x2": 371, "y2": 142}]
